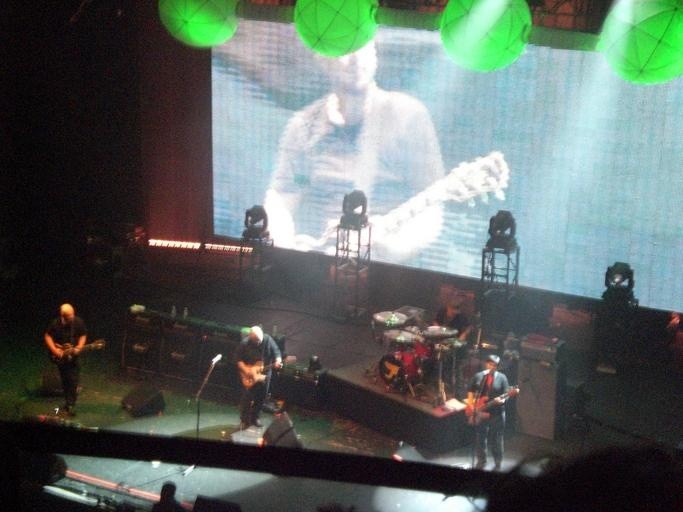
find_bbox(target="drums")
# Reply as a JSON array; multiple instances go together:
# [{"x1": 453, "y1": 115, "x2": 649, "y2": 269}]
[
  {"x1": 381, "y1": 326, "x2": 421, "y2": 356},
  {"x1": 379, "y1": 344, "x2": 433, "y2": 387},
  {"x1": 421, "y1": 326, "x2": 458, "y2": 352}
]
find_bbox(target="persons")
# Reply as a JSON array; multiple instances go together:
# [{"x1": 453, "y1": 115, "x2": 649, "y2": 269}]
[
  {"x1": 46, "y1": 304, "x2": 87, "y2": 414},
  {"x1": 237, "y1": 322, "x2": 282, "y2": 428},
  {"x1": 152, "y1": 483, "x2": 186, "y2": 512},
  {"x1": 464, "y1": 356, "x2": 505, "y2": 471},
  {"x1": 432, "y1": 297, "x2": 475, "y2": 341},
  {"x1": 665, "y1": 311, "x2": 681, "y2": 330},
  {"x1": 486, "y1": 447, "x2": 683, "y2": 512},
  {"x1": 262, "y1": 38, "x2": 445, "y2": 264}
]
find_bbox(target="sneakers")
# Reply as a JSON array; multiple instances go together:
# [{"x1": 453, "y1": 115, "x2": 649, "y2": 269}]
[{"x1": 64, "y1": 402, "x2": 75, "y2": 416}]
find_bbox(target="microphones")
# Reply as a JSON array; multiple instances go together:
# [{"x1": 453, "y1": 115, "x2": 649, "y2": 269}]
[
  {"x1": 212, "y1": 354, "x2": 222, "y2": 362},
  {"x1": 482, "y1": 369, "x2": 490, "y2": 375}
]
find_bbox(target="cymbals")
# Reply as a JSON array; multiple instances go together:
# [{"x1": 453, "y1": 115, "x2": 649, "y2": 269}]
[
  {"x1": 372, "y1": 312, "x2": 408, "y2": 326},
  {"x1": 427, "y1": 324, "x2": 458, "y2": 335}
]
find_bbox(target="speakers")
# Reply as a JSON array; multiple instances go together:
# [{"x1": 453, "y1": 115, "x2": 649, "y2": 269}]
[
  {"x1": 264, "y1": 411, "x2": 297, "y2": 448},
  {"x1": 122, "y1": 384, "x2": 166, "y2": 416},
  {"x1": 192, "y1": 495, "x2": 241, "y2": 512},
  {"x1": 42, "y1": 368, "x2": 80, "y2": 396}
]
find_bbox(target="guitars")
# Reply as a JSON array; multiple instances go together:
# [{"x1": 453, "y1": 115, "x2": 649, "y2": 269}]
[
  {"x1": 47, "y1": 338, "x2": 106, "y2": 365},
  {"x1": 465, "y1": 384, "x2": 521, "y2": 426},
  {"x1": 239, "y1": 356, "x2": 298, "y2": 389},
  {"x1": 302, "y1": 151, "x2": 509, "y2": 268}
]
